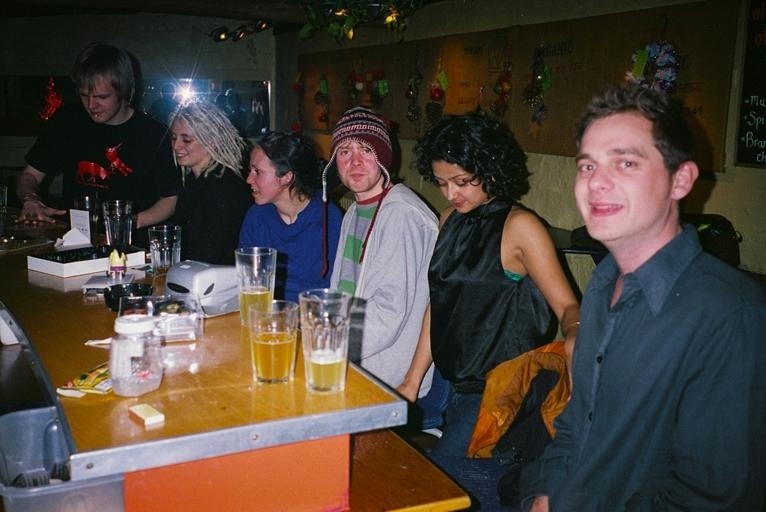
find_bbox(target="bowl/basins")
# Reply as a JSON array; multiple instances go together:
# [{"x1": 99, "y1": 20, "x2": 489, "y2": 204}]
[{"x1": 104, "y1": 284, "x2": 153, "y2": 311}]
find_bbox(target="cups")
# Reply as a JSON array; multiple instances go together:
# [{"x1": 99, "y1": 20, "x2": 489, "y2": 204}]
[
  {"x1": 0, "y1": 183, "x2": 8, "y2": 206},
  {"x1": 103, "y1": 201, "x2": 133, "y2": 246},
  {"x1": 234, "y1": 247, "x2": 277, "y2": 326},
  {"x1": 148, "y1": 226, "x2": 183, "y2": 278},
  {"x1": 299, "y1": 290, "x2": 351, "y2": 393},
  {"x1": 74, "y1": 197, "x2": 103, "y2": 240},
  {"x1": 249, "y1": 300, "x2": 299, "y2": 382}
]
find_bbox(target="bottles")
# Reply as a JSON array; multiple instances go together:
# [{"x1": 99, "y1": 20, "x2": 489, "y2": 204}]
[{"x1": 111, "y1": 315, "x2": 163, "y2": 397}]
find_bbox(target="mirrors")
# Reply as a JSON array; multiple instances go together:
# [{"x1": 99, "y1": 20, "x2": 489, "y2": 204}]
[{"x1": 141, "y1": 80, "x2": 271, "y2": 140}]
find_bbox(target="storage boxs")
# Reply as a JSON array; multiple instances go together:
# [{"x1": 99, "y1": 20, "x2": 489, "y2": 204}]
[{"x1": 0, "y1": 407, "x2": 125, "y2": 512}]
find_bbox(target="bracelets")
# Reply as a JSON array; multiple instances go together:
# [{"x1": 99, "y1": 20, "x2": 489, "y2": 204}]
[
  {"x1": 560, "y1": 321, "x2": 581, "y2": 334},
  {"x1": 21, "y1": 191, "x2": 40, "y2": 203}
]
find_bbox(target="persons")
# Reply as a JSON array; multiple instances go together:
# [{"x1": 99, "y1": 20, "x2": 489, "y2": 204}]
[
  {"x1": 395, "y1": 108, "x2": 585, "y2": 512},
  {"x1": 521, "y1": 79, "x2": 766, "y2": 510},
  {"x1": 236, "y1": 130, "x2": 346, "y2": 333},
  {"x1": 167, "y1": 101, "x2": 255, "y2": 267},
  {"x1": 146, "y1": 85, "x2": 269, "y2": 138},
  {"x1": 13, "y1": 44, "x2": 185, "y2": 252},
  {"x1": 319, "y1": 105, "x2": 442, "y2": 441}
]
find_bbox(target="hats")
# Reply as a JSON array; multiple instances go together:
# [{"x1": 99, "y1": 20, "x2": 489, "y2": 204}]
[{"x1": 322, "y1": 106, "x2": 392, "y2": 203}]
